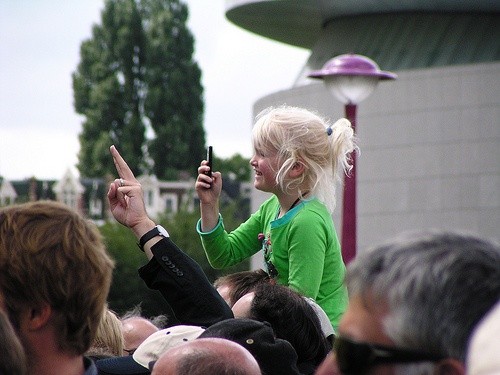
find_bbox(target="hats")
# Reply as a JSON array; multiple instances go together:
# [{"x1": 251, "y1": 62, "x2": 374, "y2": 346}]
[
  {"x1": 149, "y1": 319, "x2": 301, "y2": 375},
  {"x1": 304, "y1": 296, "x2": 337, "y2": 342},
  {"x1": 97, "y1": 325, "x2": 209, "y2": 375}
]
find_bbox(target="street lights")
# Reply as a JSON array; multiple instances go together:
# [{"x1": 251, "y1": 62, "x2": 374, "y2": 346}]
[{"x1": 306, "y1": 52, "x2": 396, "y2": 264}]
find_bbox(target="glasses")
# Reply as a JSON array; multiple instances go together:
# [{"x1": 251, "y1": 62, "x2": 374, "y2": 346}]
[{"x1": 335, "y1": 334, "x2": 453, "y2": 375}]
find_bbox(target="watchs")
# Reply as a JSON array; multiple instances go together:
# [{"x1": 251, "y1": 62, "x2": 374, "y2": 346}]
[{"x1": 136, "y1": 225, "x2": 169, "y2": 252}]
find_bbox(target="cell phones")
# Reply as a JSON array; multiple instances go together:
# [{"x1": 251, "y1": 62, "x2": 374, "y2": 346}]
[{"x1": 206, "y1": 146, "x2": 212, "y2": 179}]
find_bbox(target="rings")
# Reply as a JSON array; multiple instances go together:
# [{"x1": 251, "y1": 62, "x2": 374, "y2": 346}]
[{"x1": 120, "y1": 179, "x2": 124, "y2": 186}]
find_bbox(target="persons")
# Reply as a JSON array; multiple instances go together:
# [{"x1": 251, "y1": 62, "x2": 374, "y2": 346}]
[
  {"x1": 195, "y1": 104, "x2": 355, "y2": 333},
  {"x1": 0, "y1": 145, "x2": 500, "y2": 375}
]
